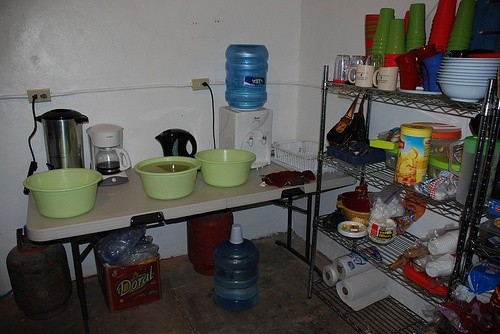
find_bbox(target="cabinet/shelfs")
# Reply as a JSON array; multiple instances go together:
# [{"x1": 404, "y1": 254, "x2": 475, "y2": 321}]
[{"x1": 308, "y1": 66, "x2": 500, "y2": 334}]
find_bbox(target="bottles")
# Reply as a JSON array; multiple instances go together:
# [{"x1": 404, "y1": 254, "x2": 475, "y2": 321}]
[
  {"x1": 224, "y1": 43, "x2": 270, "y2": 108},
  {"x1": 212, "y1": 224, "x2": 260, "y2": 311},
  {"x1": 412, "y1": 122, "x2": 461, "y2": 154}
]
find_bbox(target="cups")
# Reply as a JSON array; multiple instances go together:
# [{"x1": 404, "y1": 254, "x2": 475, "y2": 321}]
[
  {"x1": 428, "y1": 0, "x2": 476, "y2": 54},
  {"x1": 412, "y1": 226, "x2": 470, "y2": 278},
  {"x1": 348, "y1": 64, "x2": 375, "y2": 88},
  {"x1": 367, "y1": 3, "x2": 442, "y2": 92},
  {"x1": 334, "y1": 54, "x2": 352, "y2": 83},
  {"x1": 350, "y1": 55, "x2": 366, "y2": 82},
  {"x1": 369, "y1": 219, "x2": 397, "y2": 245}
]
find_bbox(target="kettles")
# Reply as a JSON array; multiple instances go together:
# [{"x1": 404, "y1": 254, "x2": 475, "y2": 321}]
[{"x1": 34, "y1": 108, "x2": 89, "y2": 167}]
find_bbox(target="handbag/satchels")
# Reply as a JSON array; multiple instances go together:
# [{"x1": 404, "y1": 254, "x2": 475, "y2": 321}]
[{"x1": 326, "y1": 92, "x2": 368, "y2": 143}]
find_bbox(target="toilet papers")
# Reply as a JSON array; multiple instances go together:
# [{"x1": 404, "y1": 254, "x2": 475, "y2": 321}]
[{"x1": 321, "y1": 246, "x2": 392, "y2": 312}]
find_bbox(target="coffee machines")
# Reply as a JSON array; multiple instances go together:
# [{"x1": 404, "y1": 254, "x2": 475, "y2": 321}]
[{"x1": 83, "y1": 123, "x2": 132, "y2": 185}]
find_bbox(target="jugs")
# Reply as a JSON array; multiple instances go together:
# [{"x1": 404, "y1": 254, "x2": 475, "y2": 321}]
[
  {"x1": 95, "y1": 148, "x2": 131, "y2": 175},
  {"x1": 155, "y1": 128, "x2": 198, "y2": 159},
  {"x1": 455, "y1": 136, "x2": 500, "y2": 206}
]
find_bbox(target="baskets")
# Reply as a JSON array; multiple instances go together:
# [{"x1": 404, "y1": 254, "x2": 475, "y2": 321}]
[{"x1": 337, "y1": 199, "x2": 371, "y2": 227}]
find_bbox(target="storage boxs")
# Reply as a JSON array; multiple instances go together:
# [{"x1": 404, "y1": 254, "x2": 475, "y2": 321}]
[{"x1": 96, "y1": 244, "x2": 163, "y2": 316}]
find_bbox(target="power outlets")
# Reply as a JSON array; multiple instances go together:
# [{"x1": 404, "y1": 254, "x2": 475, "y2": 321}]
[
  {"x1": 27, "y1": 88, "x2": 51, "y2": 103},
  {"x1": 193, "y1": 78, "x2": 210, "y2": 90}
]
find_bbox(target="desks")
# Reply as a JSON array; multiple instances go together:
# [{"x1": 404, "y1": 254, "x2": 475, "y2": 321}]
[{"x1": 26, "y1": 155, "x2": 355, "y2": 334}]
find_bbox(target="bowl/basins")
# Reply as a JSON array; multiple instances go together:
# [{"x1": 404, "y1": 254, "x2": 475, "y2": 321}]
[
  {"x1": 134, "y1": 156, "x2": 202, "y2": 201},
  {"x1": 195, "y1": 148, "x2": 256, "y2": 188},
  {"x1": 337, "y1": 184, "x2": 373, "y2": 226},
  {"x1": 23, "y1": 168, "x2": 103, "y2": 218},
  {"x1": 436, "y1": 57, "x2": 500, "y2": 103}
]
